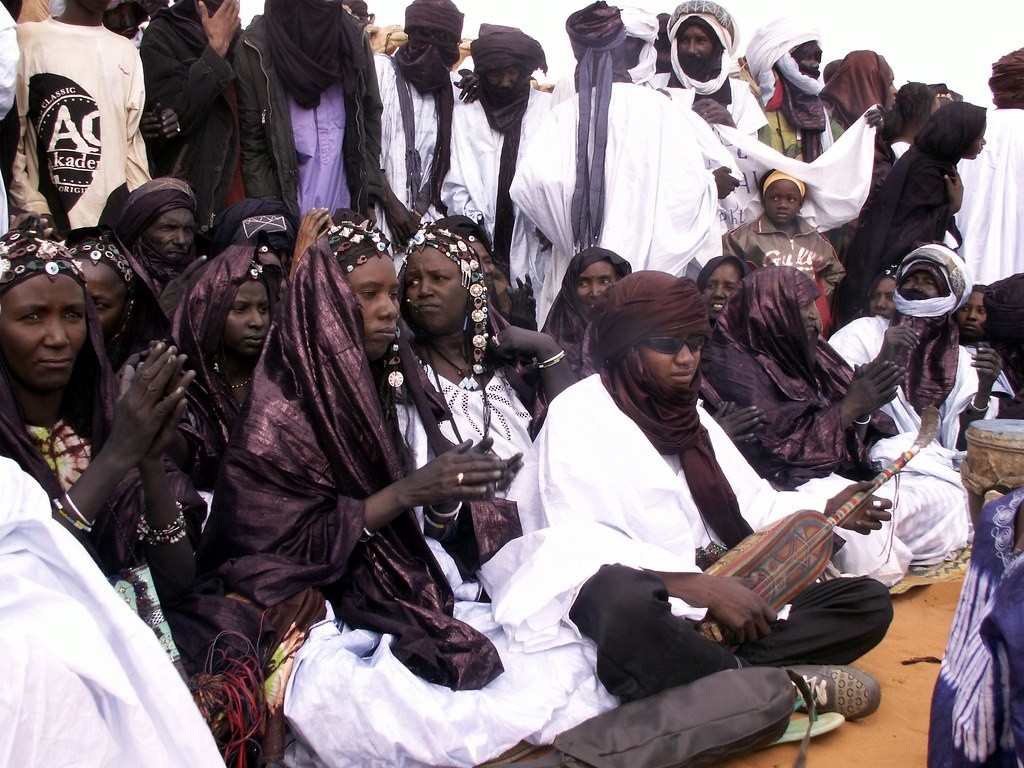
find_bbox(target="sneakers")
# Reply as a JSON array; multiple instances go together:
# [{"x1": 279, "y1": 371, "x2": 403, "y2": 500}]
[{"x1": 781, "y1": 664, "x2": 880, "y2": 721}]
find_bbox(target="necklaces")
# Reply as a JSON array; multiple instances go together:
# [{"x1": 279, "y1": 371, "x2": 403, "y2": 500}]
[
  {"x1": 231, "y1": 378, "x2": 252, "y2": 389},
  {"x1": 428, "y1": 345, "x2": 469, "y2": 376}
]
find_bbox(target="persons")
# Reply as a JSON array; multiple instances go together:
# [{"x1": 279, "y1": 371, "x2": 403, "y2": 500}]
[
  {"x1": 0, "y1": 0, "x2": 1024, "y2": 768},
  {"x1": 926, "y1": 486, "x2": 1024, "y2": 768}
]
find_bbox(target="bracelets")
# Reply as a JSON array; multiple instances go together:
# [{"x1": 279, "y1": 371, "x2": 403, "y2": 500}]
[
  {"x1": 542, "y1": 350, "x2": 565, "y2": 368},
  {"x1": 363, "y1": 527, "x2": 376, "y2": 537},
  {"x1": 854, "y1": 415, "x2": 871, "y2": 424},
  {"x1": 53, "y1": 499, "x2": 92, "y2": 532},
  {"x1": 359, "y1": 538, "x2": 370, "y2": 543},
  {"x1": 971, "y1": 396, "x2": 990, "y2": 412},
  {"x1": 424, "y1": 514, "x2": 445, "y2": 529},
  {"x1": 430, "y1": 501, "x2": 463, "y2": 517},
  {"x1": 66, "y1": 494, "x2": 95, "y2": 526},
  {"x1": 137, "y1": 500, "x2": 187, "y2": 545}
]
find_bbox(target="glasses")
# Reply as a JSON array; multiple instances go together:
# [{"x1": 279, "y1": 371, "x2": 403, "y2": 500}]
[
  {"x1": 417, "y1": 26, "x2": 451, "y2": 41},
  {"x1": 644, "y1": 336, "x2": 706, "y2": 353}
]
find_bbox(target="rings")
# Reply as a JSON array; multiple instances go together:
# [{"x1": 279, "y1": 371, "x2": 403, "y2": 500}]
[
  {"x1": 879, "y1": 117, "x2": 882, "y2": 122},
  {"x1": 177, "y1": 128, "x2": 180, "y2": 131},
  {"x1": 458, "y1": 472, "x2": 463, "y2": 485},
  {"x1": 473, "y1": 86, "x2": 477, "y2": 89}
]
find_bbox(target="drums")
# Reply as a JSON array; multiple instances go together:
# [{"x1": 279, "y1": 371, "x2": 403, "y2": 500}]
[{"x1": 960, "y1": 419, "x2": 1024, "y2": 554}]
